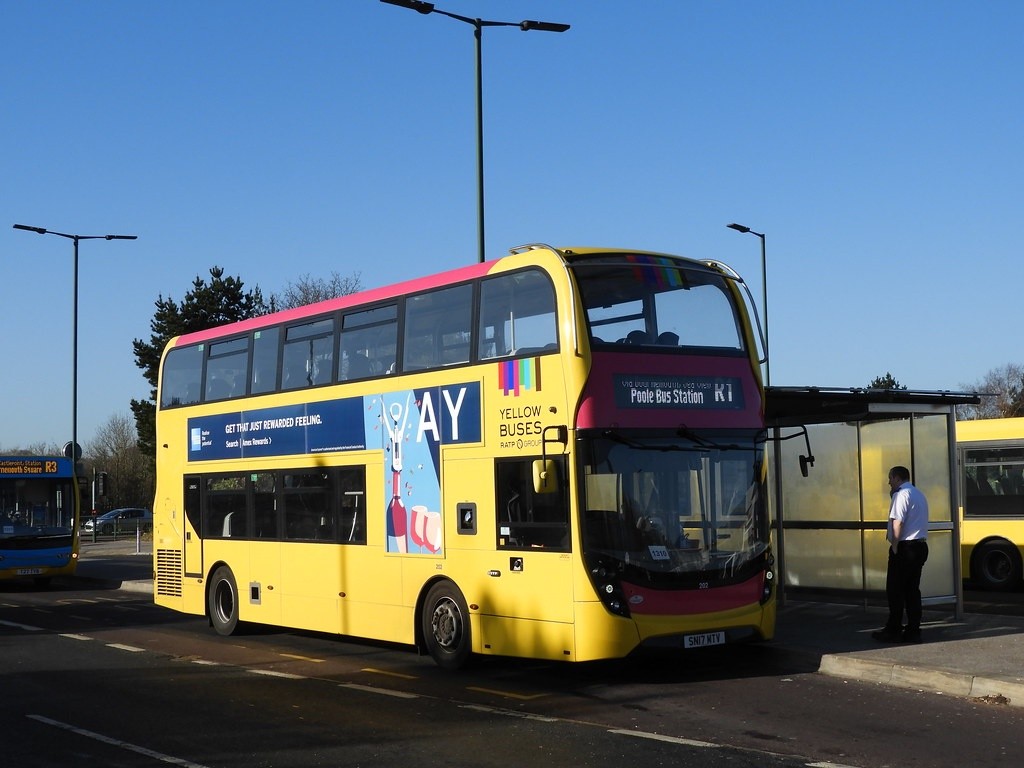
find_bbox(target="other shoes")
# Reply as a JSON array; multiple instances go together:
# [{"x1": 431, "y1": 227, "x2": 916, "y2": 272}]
[
  {"x1": 872, "y1": 626, "x2": 903, "y2": 643},
  {"x1": 903, "y1": 625, "x2": 921, "y2": 643}
]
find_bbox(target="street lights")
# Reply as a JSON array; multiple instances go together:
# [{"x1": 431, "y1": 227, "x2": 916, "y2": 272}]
[
  {"x1": 727, "y1": 222, "x2": 771, "y2": 387},
  {"x1": 13, "y1": 222, "x2": 139, "y2": 464},
  {"x1": 384, "y1": 0, "x2": 575, "y2": 269}
]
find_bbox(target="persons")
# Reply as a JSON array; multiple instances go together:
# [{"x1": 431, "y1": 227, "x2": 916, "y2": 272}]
[
  {"x1": 508, "y1": 477, "x2": 570, "y2": 549},
  {"x1": 871, "y1": 467, "x2": 929, "y2": 645},
  {"x1": 636, "y1": 496, "x2": 688, "y2": 549}
]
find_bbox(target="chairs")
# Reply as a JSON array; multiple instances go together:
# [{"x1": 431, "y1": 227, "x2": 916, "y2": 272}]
[
  {"x1": 221, "y1": 510, "x2": 244, "y2": 538},
  {"x1": 184, "y1": 329, "x2": 681, "y2": 401}
]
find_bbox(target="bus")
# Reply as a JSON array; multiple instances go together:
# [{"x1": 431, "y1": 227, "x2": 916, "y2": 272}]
[
  {"x1": 151, "y1": 246, "x2": 817, "y2": 674},
  {"x1": 0, "y1": 455, "x2": 89, "y2": 581},
  {"x1": 787, "y1": 413, "x2": 1024, "y2": 593}
]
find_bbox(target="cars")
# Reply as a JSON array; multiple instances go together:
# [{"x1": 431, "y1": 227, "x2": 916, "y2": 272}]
[{"x1": 84, "y1": 508, "x2": 153, "y2": 537}]
